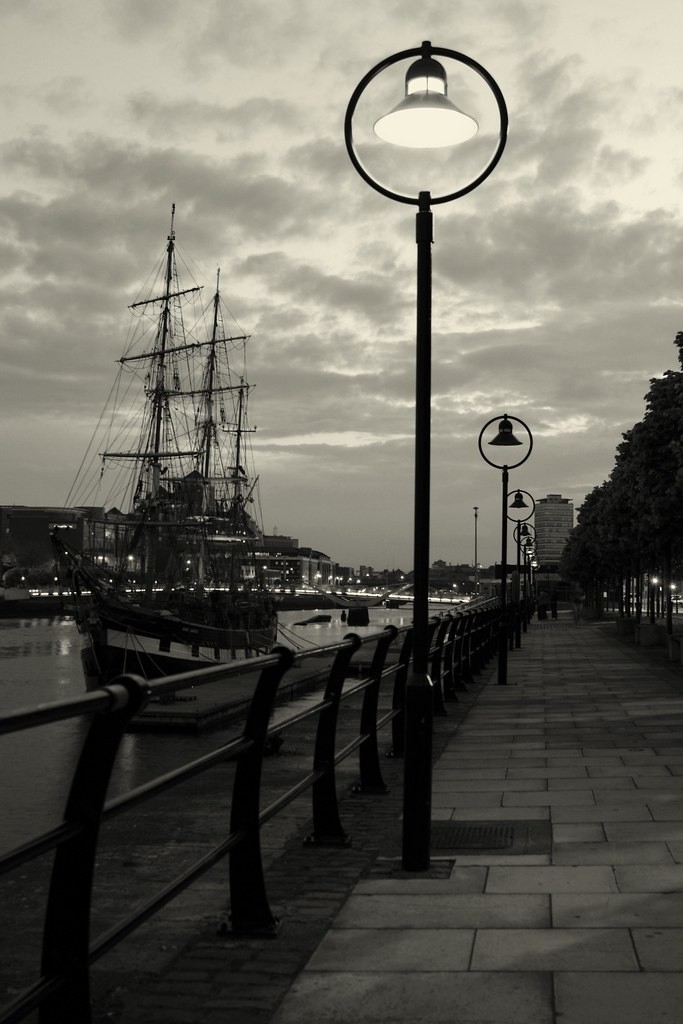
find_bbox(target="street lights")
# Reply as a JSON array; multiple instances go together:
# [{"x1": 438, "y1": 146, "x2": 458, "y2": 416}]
[
  {"x1": 507, "y1": 488, "x2": 536, "y2": 651},
  {"x1": 344, "y1": 39, "x2": 511, "y2": 872},
  {"x1": 473, "y1": 505, "x2": 480, "y2": 597},
  {"x1": 480, "y1": 412, "x2": 534, "y2": 686},
  {"x1": 514, "y1": 522, "x2": 537, "y2": 633},
  {"x1": 520, "y1": 536, "x2": 537, "y2": 626}
]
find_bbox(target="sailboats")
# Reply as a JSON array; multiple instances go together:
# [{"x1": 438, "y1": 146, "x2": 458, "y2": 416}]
[{"x1": 47, "y1": 201, "x2": 471, "y2": 734}]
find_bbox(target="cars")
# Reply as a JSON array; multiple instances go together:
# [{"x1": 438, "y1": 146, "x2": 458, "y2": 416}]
[{"x1": 669, "y1": 594, "x2": 683, "y2": 606}]
